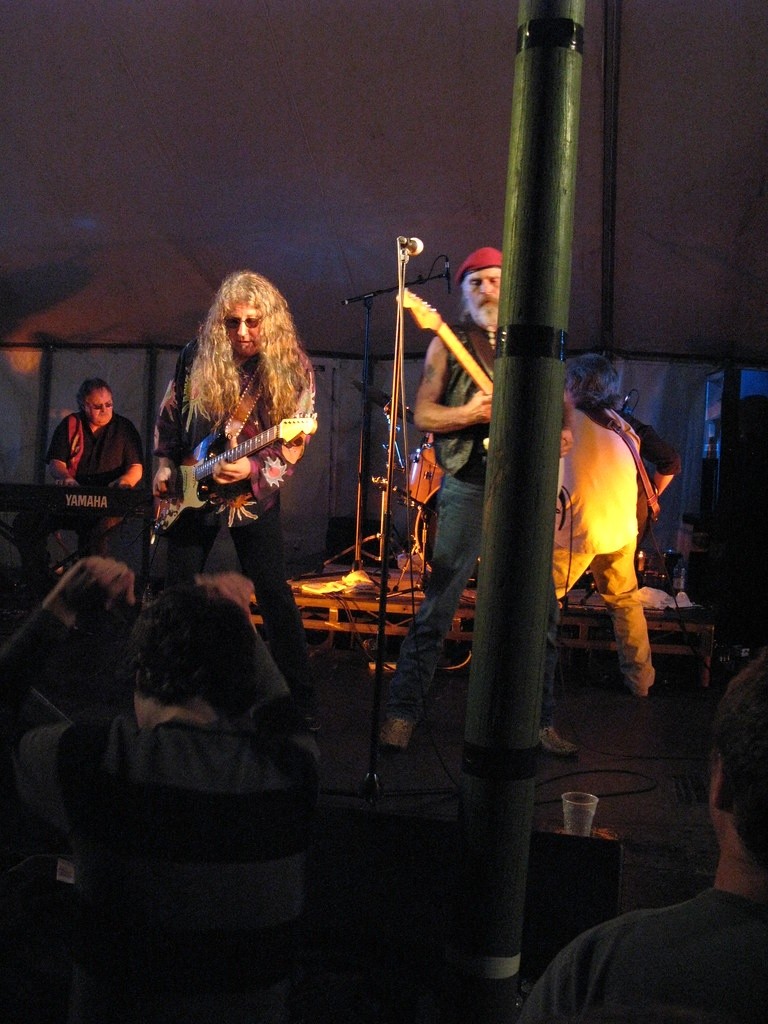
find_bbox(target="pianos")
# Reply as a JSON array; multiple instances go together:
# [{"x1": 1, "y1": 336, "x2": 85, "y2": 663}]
[{"x1": 0, "y1": 483, "x2": 151, "y2": 519}]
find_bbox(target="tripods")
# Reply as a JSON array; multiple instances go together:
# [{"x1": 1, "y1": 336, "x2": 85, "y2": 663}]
[{"x1": 323, "y1": 252, "x2": 475, "y2": 812}]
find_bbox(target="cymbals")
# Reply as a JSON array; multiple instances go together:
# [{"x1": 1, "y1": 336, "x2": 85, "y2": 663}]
[{"x1": 352, "y1": 379, "x2": 414, "y2": 425}]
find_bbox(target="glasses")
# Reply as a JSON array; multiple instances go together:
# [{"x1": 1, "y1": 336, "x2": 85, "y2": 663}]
[
  {"x1": 224, "y1": 315, "x2": 262, "y2": 329},
  {"x1": 83, "y1": 400, "x2": 114, "y2": 410}
]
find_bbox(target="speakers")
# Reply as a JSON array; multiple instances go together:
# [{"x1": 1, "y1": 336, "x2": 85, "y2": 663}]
[
  {"x1": 292, "y1": 805, "x2": 619, "y2": 978},
  {"x1": 687, "y1": 366, "x2": 768, "y2": 656}
]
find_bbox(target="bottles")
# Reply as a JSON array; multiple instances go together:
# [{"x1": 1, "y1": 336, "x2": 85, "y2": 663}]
[{"x1": 673, "y1": 558, "x2": 685, "y2": 596}]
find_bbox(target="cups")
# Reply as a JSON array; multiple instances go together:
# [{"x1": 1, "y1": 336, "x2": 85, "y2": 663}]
[{"x1": 562, "y1": 792, "x2": 599, "y2": 837}]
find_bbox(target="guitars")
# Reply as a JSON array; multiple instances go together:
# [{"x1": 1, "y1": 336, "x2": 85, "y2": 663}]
[
  {"x1": 395, "y1": 286, "x2": 567, "y2": 498},
  {"x1": 154, "y1": 412, "x2": 318, "y2": 530}
]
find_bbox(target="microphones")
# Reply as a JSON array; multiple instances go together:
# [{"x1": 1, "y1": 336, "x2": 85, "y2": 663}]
[
  {"x1": 445, "y1": 256, "x2": 451, "y2": 294},
  {"x1": 398, "y1": 236, "x2": 424, "y2": 256},
  {"x1": 622, "y1": 393, "x2": 632, "y2": 411}
]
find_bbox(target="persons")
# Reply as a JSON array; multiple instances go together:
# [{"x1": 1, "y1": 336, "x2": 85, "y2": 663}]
[
  {"x1": 149, "y1": 269, "x2": 320, "y2": 730},
  {"x1": 379, "y1": 247, "x2": 573, "y2": 766},
  {"x1": 0, "y1": 554, "x2": 323, "y2": 1024},
  {"x1": 517, "y1": 647, "x2": 767, "y2": 1023},
  {"x1": 14, "y1": 377, "x2": 144, "y2": 576},
  {"x1": 551, "y1": 356, "x2": 682, "y2": 696}
]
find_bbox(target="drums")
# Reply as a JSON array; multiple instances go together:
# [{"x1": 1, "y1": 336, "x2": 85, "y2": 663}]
[
  {"x1": 413, "y1": 484, "x2": 478, "y2": 581},
  {"x1": 398, "y1": 446, "x2": 445, "y2": 507}
]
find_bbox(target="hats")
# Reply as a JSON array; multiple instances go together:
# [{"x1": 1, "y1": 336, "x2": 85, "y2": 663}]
[{"x1": 453, "y1": 247, "x2": 503, "y2": 287}]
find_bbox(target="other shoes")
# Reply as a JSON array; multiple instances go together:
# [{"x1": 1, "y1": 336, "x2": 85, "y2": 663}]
[
  {"x1": 538, "y1": 726, "x2": 580, "y2": 757},
  {"x1": 624, "y1": 675, "x2": 649, "y2": 698},
  {"x1": 378, "y1": 713, "x2": 413, "y2": 750}
]
